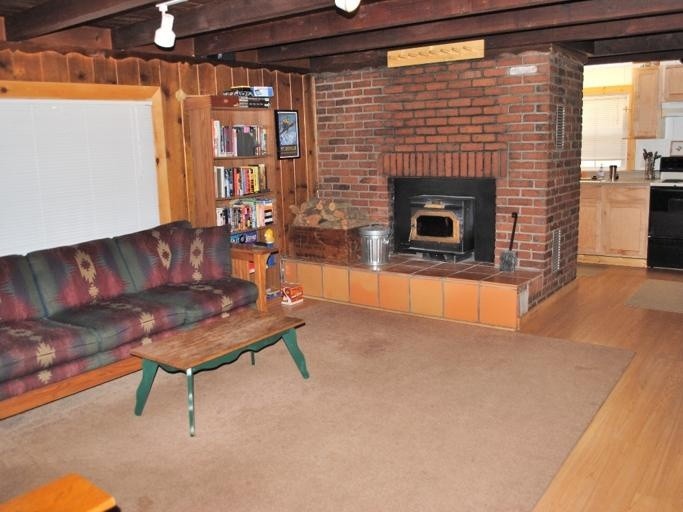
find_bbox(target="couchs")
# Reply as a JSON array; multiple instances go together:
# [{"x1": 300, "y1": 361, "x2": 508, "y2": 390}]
[{"x1": 0, "y1": 216, "x2": 261, "y2": 428}]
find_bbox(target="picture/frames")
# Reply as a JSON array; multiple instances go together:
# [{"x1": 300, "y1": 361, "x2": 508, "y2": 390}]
[{"x1": 274, "y1": 109, "x2": 302, "y2": 161}]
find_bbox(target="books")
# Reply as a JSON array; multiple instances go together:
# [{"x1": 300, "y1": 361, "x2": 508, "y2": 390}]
[{"x1": 212, "y1": 117, "x2": 275, "y2": 245}]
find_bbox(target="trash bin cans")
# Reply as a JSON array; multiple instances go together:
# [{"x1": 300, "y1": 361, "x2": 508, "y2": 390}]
[{"x1": 358, "y1": 225, "x2": 391, "y2": 265}]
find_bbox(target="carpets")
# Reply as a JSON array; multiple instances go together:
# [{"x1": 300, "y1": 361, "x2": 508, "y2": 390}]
[{"x1": 1, "y1": 298, "x2": 636, "y2": 512}]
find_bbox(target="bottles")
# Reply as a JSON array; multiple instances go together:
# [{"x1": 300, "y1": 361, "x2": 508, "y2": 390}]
[{"x1": 609, "y1": 165, "x2": 617, "y2": 180}]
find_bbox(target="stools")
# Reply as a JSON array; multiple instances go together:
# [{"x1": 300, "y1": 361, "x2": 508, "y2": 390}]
[{"x1": 0, "y1": 474, "x2": 122, "y2": 512}]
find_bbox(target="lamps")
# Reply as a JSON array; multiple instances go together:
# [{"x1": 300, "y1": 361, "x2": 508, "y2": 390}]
[
  {"x1": 153, "y1": 0, "x2": 188, "y2": 47},
  {"x1": 334, "y1": 0, "x2": 361, "y2": 13}
]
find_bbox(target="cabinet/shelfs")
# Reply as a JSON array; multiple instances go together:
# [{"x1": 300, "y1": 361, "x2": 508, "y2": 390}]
[
  {"x1": 577, "y1": 184, "x2": 652, "y2": 270},
  {"x1": 180, "y1": 88, "x2": 282, "y2": 311}
]
